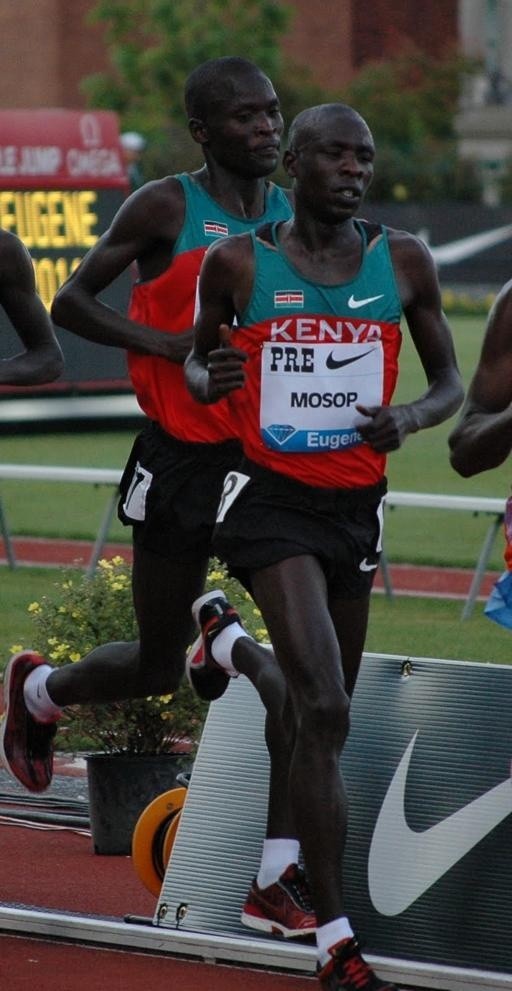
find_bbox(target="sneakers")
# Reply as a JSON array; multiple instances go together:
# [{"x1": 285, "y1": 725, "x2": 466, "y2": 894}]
[
  {"x1": 186, "y1": 590, "x2": 244, "y2": 700},
  {"x1": 241, "y1": 861, "x2": 318, "y2": 940},
  {"x1": 0, "y1": 647, "x2": 57, "y2": 793},
  {"x1": 314, "y1": 937, "x2": 391, "y2": 988}
]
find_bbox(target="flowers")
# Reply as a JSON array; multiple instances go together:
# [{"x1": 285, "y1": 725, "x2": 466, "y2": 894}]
[{"x1": 9, "y1": 556, "x2": 270, "y2": 769}]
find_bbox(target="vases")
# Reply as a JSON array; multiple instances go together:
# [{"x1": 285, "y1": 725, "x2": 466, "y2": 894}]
[{"x1": 83, "y1": 753, "x2": 194, "y2": 857}]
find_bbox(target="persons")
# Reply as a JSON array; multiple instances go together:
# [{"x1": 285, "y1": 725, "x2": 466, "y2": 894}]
[
  {"x1": 1, "y1": 229, "x2": 66, "y2": 385},
  {"x1": 448, "y1": 277, "x2": 512, "y2": 629},
  {"x1": 1, "y1": 55, "x2": 318, "y2": 945},
  {"x1": 183, "y1": 104, "x2": 466, "y2": 991}
]
